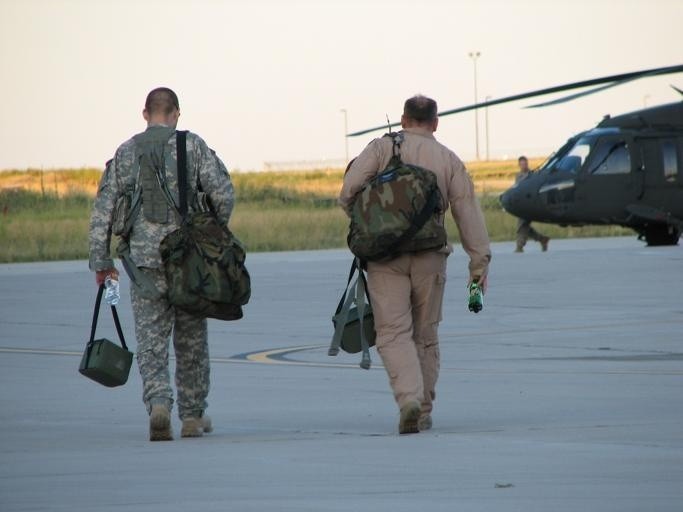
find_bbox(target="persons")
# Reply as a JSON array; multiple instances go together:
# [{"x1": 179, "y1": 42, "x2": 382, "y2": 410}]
[
  {"x1": 514, "y1": 157, "x2": 550, "y2": 252},
  {"x1": 88, "y1": 88, "x2": 235, "y2": 440},
  {"x1": 339, "y1": 95, "x2": 491, "y2": 434}
]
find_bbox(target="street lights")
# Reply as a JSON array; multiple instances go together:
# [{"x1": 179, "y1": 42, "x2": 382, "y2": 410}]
[
  {"x1": 485, "y1": 95, "x2": 491, "y2": 163},
  {"x1": 468, "y1": 51, "x2": 482, "y2": 164}
]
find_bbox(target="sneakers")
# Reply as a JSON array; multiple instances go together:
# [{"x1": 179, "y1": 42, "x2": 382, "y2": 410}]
[
  {"x1": 149, "y1": 403, "x2": 173, "y2": 441},
  {"x1": 398, "y1": 401, "x2": 431, "y2": 433},
  {"x1": 181, "y1": 413, "x2": 210, "y2": 437}
]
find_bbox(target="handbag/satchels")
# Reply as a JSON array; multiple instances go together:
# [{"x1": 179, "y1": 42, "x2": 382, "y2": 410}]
[
  {"x1": 159, "y1": 212, "x2": 251, "y2": 321},
  {"x1": 78, "y1": 339, "x2": 133, "y2": 387},
  {"x1": 332, "y1": 302, "x2": 375, "y2": 353},
  {"x1": 346, "y1": 162, "x2": 439, "y2": 261}
]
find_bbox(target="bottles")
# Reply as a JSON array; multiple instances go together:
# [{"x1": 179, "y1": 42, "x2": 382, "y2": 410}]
[
  {"x1": 104, "y1": 270, "x2": 120, "y2": 307},
  {"x1": 466, "y1": 277, "x2": 485, "y2": 313}
]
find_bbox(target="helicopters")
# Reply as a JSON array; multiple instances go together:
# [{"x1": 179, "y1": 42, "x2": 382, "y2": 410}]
[{"x1": 345, "y1": 64, "x2": 683, "y2": 245}]
